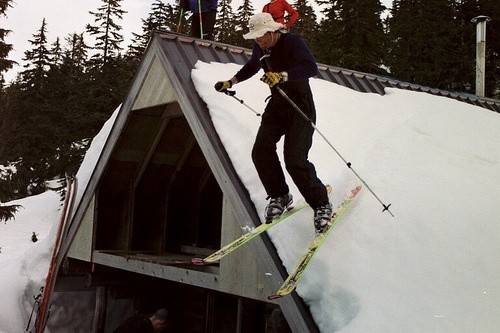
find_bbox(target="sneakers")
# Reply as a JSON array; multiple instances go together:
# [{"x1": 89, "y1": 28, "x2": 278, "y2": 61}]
[
  {"x1": 264, "y1": 192, "x2": 293, "y2": 220},
  {"x1": 314, "y1": 204, "x2": 332, "y2": 233}
]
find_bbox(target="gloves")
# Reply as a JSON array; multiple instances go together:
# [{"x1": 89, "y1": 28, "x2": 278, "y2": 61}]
[
  {"x1": 217, "y1": 80, "x2": 232, "y2": 92},
  {"x1": 262, "y1": 73, "x2": 284, "y2": 87}
]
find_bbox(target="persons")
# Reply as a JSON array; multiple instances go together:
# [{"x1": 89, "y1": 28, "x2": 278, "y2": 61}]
[
  {"x1": 214, "y1": 12, "x2": 338, "y2": 235},
  {"x1": 263, "y1": 0, "x2": 299, "y2": 29},
  {"x1": 112, "y1": 308, "x2": 177, "y2": 333},
  {"x1": 189, "y1": 0, "x2": 218, "y2": 41}
]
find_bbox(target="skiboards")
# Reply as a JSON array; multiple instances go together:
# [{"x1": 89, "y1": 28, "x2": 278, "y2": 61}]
[{"x1": 188, "y1": 181, "x2": 366, "y2": 300}]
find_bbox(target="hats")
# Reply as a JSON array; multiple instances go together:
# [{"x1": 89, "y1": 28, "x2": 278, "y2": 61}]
[{"x1": 243, "y1": 13, "x2": 283, "y2": 39}]
